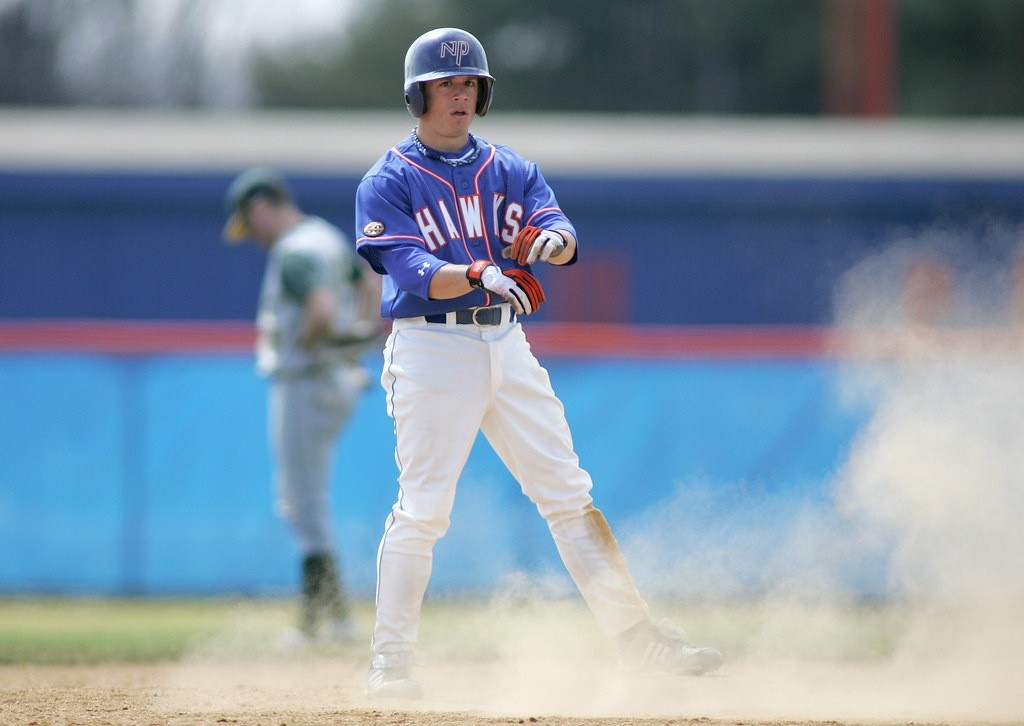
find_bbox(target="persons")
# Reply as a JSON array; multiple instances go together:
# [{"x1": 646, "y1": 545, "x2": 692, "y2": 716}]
[
  {"x1": 222, "y1": 167, "x2": 389, "y2": 657},
  {"x1": 357, "y1": 27, "x2": 722, "y2": 697}
]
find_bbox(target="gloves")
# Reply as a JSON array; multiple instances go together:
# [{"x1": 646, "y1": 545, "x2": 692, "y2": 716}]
[
  {"x1": 501, "y1": 225, "x2": 568, "y2": 267},
  {"x1": 466, "y1": 260, "x2": 546, "y2": 316}
]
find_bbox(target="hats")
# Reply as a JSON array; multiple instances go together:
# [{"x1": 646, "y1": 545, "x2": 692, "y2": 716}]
[{"x1": 224, "y1": 170, "x2": 287, "y2": 244}]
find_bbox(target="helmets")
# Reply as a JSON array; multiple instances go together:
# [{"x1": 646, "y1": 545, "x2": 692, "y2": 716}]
[{"x1": 403, "y1": 28, "x2": 495, "y2": 119}]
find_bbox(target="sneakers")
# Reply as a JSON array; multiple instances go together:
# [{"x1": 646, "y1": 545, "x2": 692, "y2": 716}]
[
  {"x1": 622, "y1": 619, "x2": 723, "y2": 680},
  {"x1": 367, "y1": 649, "x2": 422, "y2": 699}
]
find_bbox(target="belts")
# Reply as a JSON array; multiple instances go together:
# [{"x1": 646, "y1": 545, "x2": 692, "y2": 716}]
[{"x1": 425, "y1": 305, "x2": 515, "y2": 328}]
